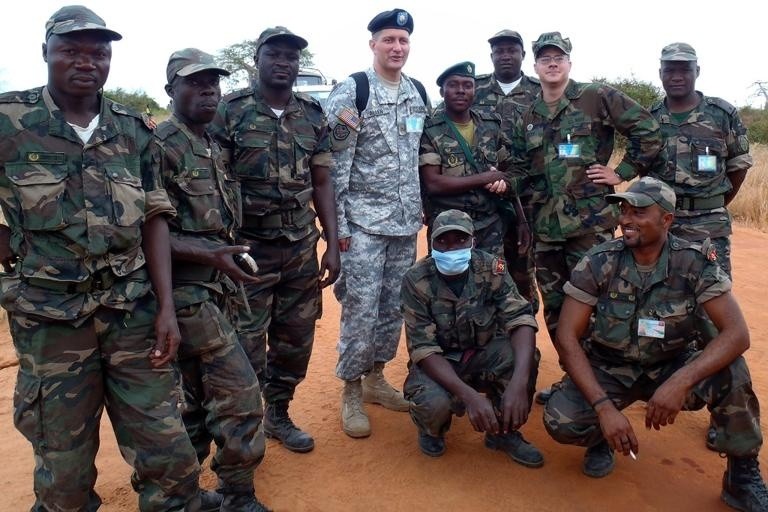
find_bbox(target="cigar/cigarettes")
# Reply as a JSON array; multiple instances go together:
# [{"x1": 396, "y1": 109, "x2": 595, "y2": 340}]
[{"x1": 627, "y1": 448, "x2": 638, "y2": 462}]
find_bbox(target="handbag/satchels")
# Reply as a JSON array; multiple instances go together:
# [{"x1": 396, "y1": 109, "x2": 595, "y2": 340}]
[{"x1": 494, "y1": 195, "x2": 518, "y2": 225}]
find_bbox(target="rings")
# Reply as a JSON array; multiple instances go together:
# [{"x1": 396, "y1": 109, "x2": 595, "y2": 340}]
[{"x1": 622, "y1": 441, "x2": 628, "y2": 445}]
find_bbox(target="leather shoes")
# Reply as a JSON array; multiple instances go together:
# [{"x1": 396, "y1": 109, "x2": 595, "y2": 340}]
[
  {"x1": 534, "y1": 389, "x2": 551, "y2": 405},
  {"x1": 185, "y1": 488, "x2": 223, "y2": 512},
  {"x1": 706, "y1": 421, "x2": 727, "y2": 453}
]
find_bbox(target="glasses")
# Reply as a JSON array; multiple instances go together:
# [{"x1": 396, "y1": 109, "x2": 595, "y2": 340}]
[{"x1": 537, "y1": 56, "x2": 568, "y2": 66}]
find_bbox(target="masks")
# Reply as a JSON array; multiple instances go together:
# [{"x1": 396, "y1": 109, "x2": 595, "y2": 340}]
[{"x1": 430, "y1": 248, "x2": 472, "y2": 276}]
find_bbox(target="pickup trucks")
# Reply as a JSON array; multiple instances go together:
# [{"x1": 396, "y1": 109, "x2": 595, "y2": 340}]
[{"x1": 292, "y1": 68, "x2": 346, "y2": 111}]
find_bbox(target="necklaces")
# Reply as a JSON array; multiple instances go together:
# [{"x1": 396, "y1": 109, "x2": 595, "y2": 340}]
[{"x1": 271, "y1": 101, "x2": 287, "y2": 107}]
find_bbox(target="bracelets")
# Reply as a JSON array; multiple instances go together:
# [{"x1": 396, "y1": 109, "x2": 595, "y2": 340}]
[
  {"x1": 517, "y1": 221, "x2": 529, "y2": 224},
  {"x1": 614, "y1": 173, "x2": 620, "y2": 178},
  {"x1": 592, "y1": 397, "x2": 609, "y2": 410}
]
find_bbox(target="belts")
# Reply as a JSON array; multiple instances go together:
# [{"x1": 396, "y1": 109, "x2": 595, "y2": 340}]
[
  {"x1": 678, "y1": 195, "x2": 725, "y2": 211},
  {"x1": 242, "y1": 203, "x2": 310, "y2": 230}
]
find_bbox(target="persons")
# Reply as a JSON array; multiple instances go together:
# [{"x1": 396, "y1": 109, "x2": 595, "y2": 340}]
[
  {"x1": 207, "y1": 27, "x2": 340, "y2": 453},
  {"x1": 418, "y1": 61, "x2": 532, "y2": 260},
  {"x1": 542, "y1": 176, "x2": 768, "y2": 511},
  {"x1": 1, "y1": 6, "x2": 203, "y2": 512},
  {"x1": 625, "y1": 44, "x2": 754, "y2": 321},
  {"x1": 323, "y1": 8, "x2": 432, "y2": 439},
  {"x1": 430, "y1": 30, "x2": 541, "y2": 320},
  {"x1": 483, "y1": 32, "x2": 663, "y2": 406},
  {"x1": 400, "y1": 210, "x2": 545, "y2": 468},
  {"x1": 132, "y1": 47, "x2": 274, "y2": 511}
]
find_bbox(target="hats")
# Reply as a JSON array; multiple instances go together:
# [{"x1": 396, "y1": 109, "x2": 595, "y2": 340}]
[
  {"x1": 659, "y1": 43, "x2": 697, "y2": 63},
  {"x1": 532, "y1": 32, "x2": 572, "y2": 62},
  {"x1": 431, "y1": 209, "x2": 475, "y2": 240},
  {"x1": 487, "y1": 29, "x2": 523, "y2": 50},
  {"x1": 45, "y1": 5, "x2": 122, "y2": 43},
  {"x1": 604, "y1": 176, "x2": 677, "y2": 213},
  {"x1": 368, "y1": 9, "x2": 414, "y2": 34},
  {"x1": 166, "y1": 49, "x2": 231, "y2": 84},
  {"x1": 436, "y1": 62, "x2": 475, "y2": 87},
  {"x1": 255, "y1": 26, "x2": 308, "y2": 58}
]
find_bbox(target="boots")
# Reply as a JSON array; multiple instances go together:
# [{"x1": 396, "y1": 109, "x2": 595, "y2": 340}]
[
  {"x1": 418, "y1": 428, "x2": 446, "y2": 457},
  {"x1": 484, "y1": 406, "x2": 544, "y2": 468},
  {"x1": 584, "y1": 438, "x2": 615, "y2": 477},
  {"x1": 720, "y1": 455, "x2": 767, "y2": 512},
  {"x1": 261, "y1": 399, "x2": 314, "y2": 453},
  {"x1": 216, "y1": 479, "x2": 272, "y2": 512},
  {"x1": 341, "y1": 377, "x2": 371, "y2": 438},
  {"x1": 361, "y1": 362, "x2": 409, "y2": 412}
]
